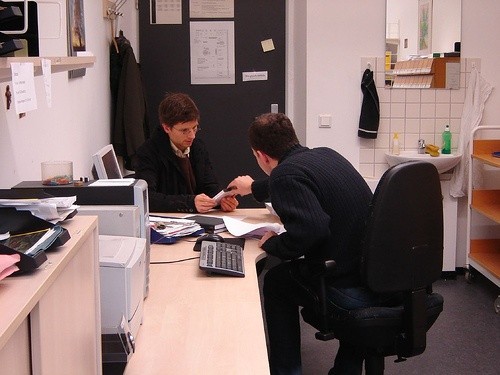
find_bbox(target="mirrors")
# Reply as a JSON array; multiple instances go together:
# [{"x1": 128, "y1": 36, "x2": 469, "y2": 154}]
[{"x1": 384, "y1": 0, "x2": 462, "y2": 90}]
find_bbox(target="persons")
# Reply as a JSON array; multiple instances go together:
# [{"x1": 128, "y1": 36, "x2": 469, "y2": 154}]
[
  {"x1": 228, "y1": 113, "x2": 374, "y2": 375},
  {"x1": 129, "y1": 94, "x2": 239, "y2": 213}
]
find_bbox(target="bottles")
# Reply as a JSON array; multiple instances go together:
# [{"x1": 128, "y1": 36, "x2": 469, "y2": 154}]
[
  {"x1": 441, "y1": 125, "x2": 451, "y2": 154},
  {"x1": 393, "y1": 132, "x2": 399, "y2": 154}
]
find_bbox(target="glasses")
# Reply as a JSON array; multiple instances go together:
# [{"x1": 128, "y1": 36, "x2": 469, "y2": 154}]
[{"x1": 173, "y1": 123, "x2": 200, "y2": 135}]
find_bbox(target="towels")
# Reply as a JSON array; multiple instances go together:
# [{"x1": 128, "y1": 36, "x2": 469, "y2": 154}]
[{"x1": 356, "y1": 68, "x2": 380, "y2": 140}]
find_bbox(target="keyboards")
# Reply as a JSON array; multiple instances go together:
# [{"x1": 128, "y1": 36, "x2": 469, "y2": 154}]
[{"x1": 199, "y1": 241, "x2": 245, "y2": 277}]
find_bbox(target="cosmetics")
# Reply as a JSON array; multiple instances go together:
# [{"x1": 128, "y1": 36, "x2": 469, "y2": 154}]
[{"x1": 392, "y1": 133, "x2": 400, "y2": 155}]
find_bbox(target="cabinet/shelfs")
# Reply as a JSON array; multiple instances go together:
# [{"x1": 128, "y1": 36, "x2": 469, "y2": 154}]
[
  {"x1": 0, "y1": 214, "x2": 103, "y2": 375},
  {"x1": 465, "y1": 125, "x2": 500, "y2": 313}
]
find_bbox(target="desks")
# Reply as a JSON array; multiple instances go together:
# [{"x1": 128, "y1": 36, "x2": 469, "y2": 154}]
[{"x1": 123, "y1": 208, "x2": 285, "y2": 375}]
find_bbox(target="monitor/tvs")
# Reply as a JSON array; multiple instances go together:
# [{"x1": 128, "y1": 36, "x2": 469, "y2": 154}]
[{"x1": 91, "y1": 144, "x2": 123, "y2": 180}]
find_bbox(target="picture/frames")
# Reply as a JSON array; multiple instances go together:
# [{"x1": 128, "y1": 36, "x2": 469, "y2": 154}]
[{"x1": 417, "y1": 0, "x2": 433, "y2": 55}]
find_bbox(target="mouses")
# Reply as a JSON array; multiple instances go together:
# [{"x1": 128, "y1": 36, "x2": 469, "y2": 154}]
[{"x1": 193, "y1": 235, "x2": 224, "y2": 251}]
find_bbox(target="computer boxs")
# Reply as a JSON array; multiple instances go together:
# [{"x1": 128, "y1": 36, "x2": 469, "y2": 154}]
[{"x1": 11, "y1": 177, "x2": 151, "y2": 299}]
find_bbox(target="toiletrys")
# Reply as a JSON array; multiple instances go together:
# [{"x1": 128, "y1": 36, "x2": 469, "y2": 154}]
[
  {"x1": 441, "y1": 124, "x2": 451, "y2": 154},
  {"x1": 393, "y1": 130, "x2": 400, "y2": 155}
]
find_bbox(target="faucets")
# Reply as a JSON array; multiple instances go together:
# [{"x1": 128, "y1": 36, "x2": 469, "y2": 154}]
[{"x1": 418, "y1": 138, "x2": 425, "y2": 154}]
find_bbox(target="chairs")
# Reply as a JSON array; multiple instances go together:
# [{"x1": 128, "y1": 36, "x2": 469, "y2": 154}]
[{"x1": 300, "y1": 159, "x2": 444, "y2": 375}]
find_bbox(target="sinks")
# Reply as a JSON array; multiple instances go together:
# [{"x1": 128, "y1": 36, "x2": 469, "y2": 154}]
[{"x1": 384, "y1": 152, "x2": 462, "y2": 173}]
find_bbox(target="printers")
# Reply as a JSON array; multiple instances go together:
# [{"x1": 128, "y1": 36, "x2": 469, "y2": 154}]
[{"x1": 98, "y1": 234, "x2": 146, "y2": 338}]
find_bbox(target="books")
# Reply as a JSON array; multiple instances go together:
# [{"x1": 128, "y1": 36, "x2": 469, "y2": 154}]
[{"x1": 212, "y1": 188, "x2": 235, "y2": 208}]
